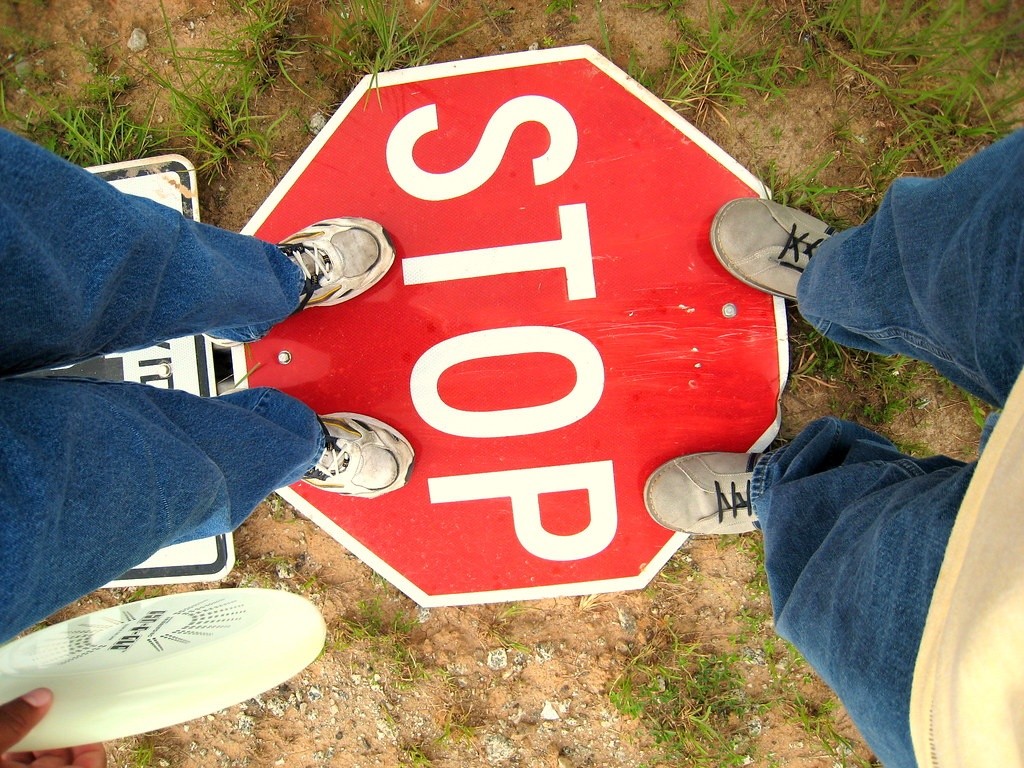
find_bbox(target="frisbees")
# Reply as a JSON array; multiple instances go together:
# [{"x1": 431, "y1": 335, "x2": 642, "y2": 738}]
[{"x1": 0, "y1": 588, "x2": 327, "y2": 756}]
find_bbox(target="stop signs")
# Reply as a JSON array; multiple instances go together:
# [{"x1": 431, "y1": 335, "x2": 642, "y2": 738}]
[{"x1": 229, "y1": 40, "x2": 793, "y2": 609}]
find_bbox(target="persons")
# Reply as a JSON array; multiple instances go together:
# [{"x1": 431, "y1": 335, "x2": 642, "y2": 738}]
[
  {"x1": 0, "y1": 127, "x2": 415, "y2": 768},
  {"x1": 642, "y1": 129, "x2": 1024, "y2": 768}
]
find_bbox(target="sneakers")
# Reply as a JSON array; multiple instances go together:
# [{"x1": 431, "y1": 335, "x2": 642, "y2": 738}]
[
  {"x1": 300, "y1": 412, "x2": 415, "y2": 497},
  {"x1": 201, "y1": 216, "x2": 396, "y2": 348},
  {"x1": 643, "y1": 452, "x2": 771, "y2": 536},
  {"x1": 710, "y1": 198, "x2": 844, "y2": 303}
]
